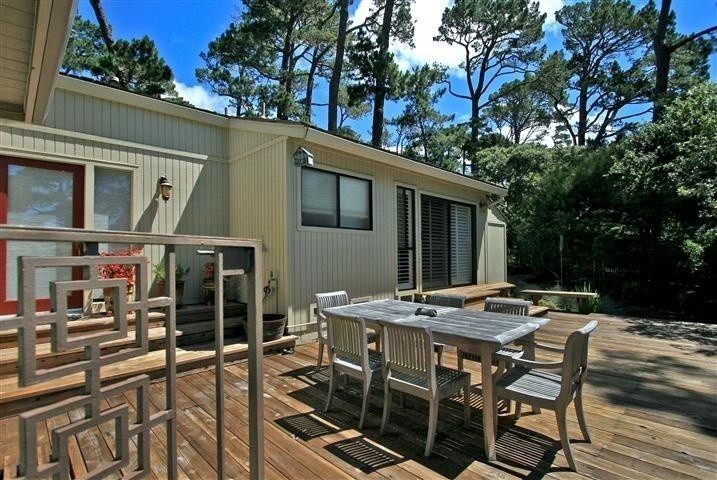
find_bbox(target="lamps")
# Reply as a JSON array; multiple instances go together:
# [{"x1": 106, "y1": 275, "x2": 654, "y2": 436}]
[{"x1": 160, "y1": 175, "x2": 172, "y2": 204}]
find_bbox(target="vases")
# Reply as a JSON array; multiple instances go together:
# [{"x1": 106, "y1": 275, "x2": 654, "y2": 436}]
[
  {"x1": 102, "y1": 284, "x2": 131, "y2": 313},
  {"x1": 199, "y1": 281, "x2": 225, "y2": 304}
]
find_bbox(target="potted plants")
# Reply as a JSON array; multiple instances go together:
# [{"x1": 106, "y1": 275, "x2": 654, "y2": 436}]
[{"x1": 150, "y1": 257, "x2": 190, "y2": 308}]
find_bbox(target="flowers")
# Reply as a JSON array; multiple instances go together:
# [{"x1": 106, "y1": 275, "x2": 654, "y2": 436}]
[
  {"x1": 200, "y1": 261, "x2": 215, "y2": 280},
  {"x1": 94, "y1": 245, "x2": 149, "y2": 287}
]
